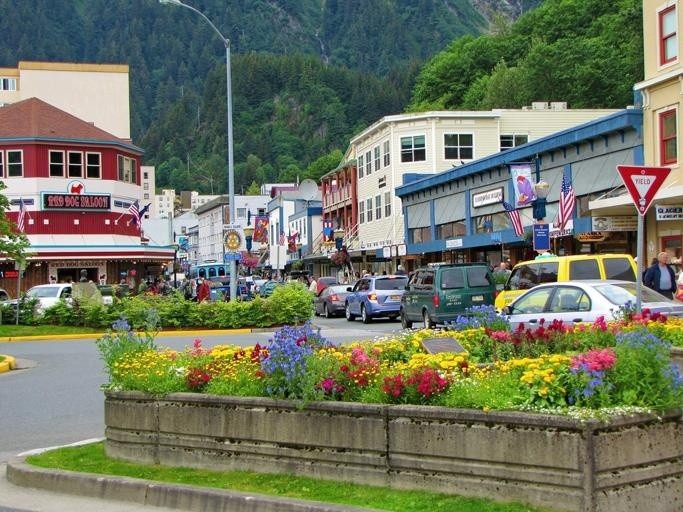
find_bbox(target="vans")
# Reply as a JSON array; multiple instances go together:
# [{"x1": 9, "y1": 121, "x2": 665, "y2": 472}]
[
  {"x1": 399, "y1": 262, "x2": 500, "y2": 330},
  {"x1": 494, "y1": 253, "x2": 638, "y2": 316}
]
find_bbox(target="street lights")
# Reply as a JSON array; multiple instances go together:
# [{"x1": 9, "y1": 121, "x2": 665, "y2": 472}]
[{"x1": 159, "y1": 0, "x2": 239, "y2": 304}]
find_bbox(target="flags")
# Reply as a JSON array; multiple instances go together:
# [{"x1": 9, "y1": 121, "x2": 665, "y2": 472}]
[
  {"x1": 501, "y1": 201, "x2": 524, "y2": 236},
  {"x1": 557, "y1": 173, "x2": 577, "y2": 230},
  {"x1": 17, "y1": 199, "x2": 25, "y2": 233},
  {"x1": 322, "y1": 221, "x2": 333, "y2": 243},
  {"x1": 130, "y1": 203, "x2": 140, "y2": 224},
  {"x1": 280, "y1": 229, "x2": 285, "y2": 246},
  {"x1": 133, "y1": 205, "x2": 148, "y2": 224}
]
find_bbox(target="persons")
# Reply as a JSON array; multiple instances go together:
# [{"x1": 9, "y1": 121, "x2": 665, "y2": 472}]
[
  {"x1": 645, "y1": 251, "x2": 677, "y2": 301},
  {"x1": 238, "y1": 267, "x2": 317, "y2": 291},
  {"x1": 50, "y1": 270, "x2": 210, "y2": 303},
  {"x1": 491, "y1": 259, "x2": 513, "y2": 276},
  {"x1": 642, "y1": 258, "x2": 658, "y2": 291},
  {"x1": 338, "y1": 265, "x2": 403, "y2": 284}
]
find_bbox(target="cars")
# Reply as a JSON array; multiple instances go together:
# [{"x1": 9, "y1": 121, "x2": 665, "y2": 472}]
[
  {"x1": 344, "y1": 275, "x2": 410, "y2": 324},
  {"x1": 208, "y1": 274, "x2": 353, "y2": 318},
  {"x1": 0, "y1": 283, "x2": 114, "y2": 318},
  {"x1": 496, "y1": 278, "x2": 683, "y2": 337}
]
